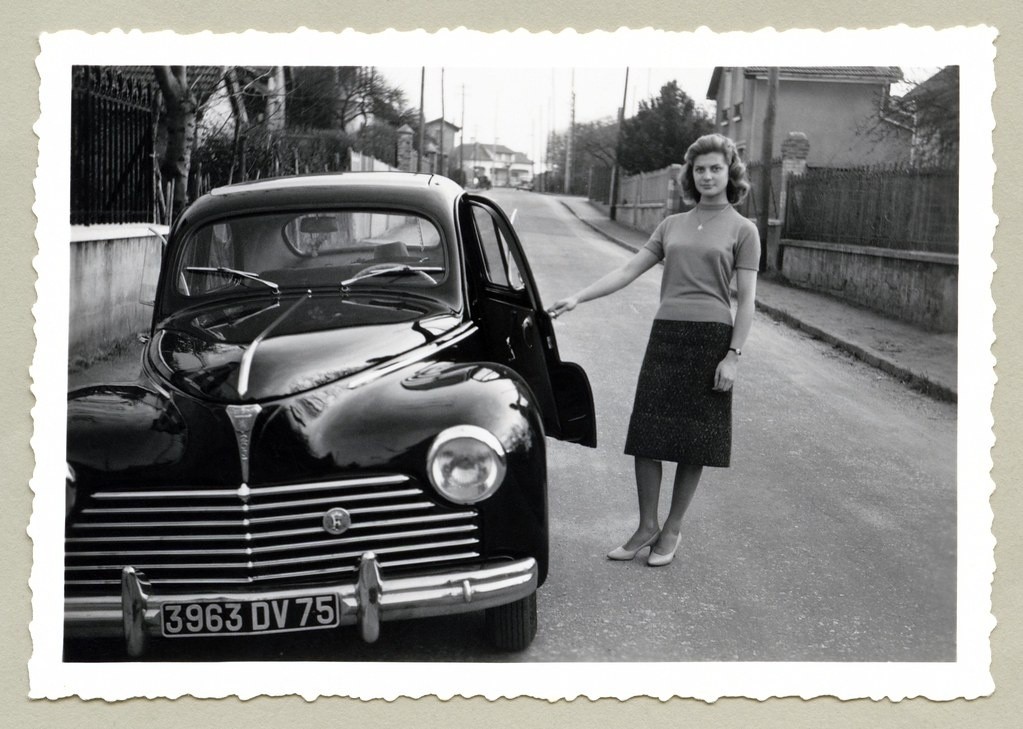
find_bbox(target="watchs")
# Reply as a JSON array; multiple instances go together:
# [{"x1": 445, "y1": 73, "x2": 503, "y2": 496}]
[{"x1": 728, "y1": 346, "x2": 742, "y2": 357}]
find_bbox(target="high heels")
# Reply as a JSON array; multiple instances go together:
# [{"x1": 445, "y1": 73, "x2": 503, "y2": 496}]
[
  {"x1": 607, "y1": 526, "x2": 662, "y2": 560},
  {"x1": 648, "y1": 532, "x2": 682, "y2": 566}
]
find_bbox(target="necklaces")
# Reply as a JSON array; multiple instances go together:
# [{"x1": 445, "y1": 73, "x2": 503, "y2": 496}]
[{"x1": 696, "y1": 202, "x2": 732, "y2": 231}]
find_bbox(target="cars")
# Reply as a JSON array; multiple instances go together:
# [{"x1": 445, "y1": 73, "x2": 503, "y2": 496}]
[{"x1": 68, "y1": 173, "x2": 597, "y2": 666}]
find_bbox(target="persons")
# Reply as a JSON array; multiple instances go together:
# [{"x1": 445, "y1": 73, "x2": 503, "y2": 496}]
[
  {"x1": 509, "y1": 387, "x2": 532, "y2": 430},
  {"x1": 540, "y1": 131, "x2": 762, "y2": 567}
]
